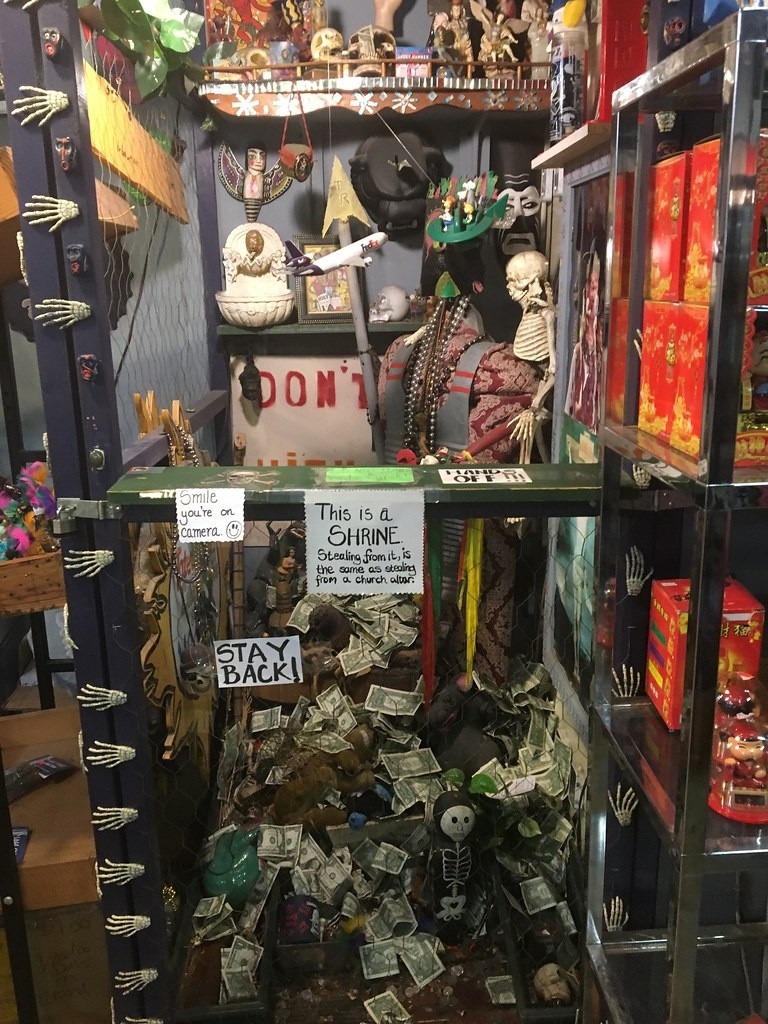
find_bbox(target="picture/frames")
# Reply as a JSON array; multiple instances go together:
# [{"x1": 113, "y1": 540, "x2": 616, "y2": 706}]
[{"x1": 292, "y1": 232, "x2": 369, "y2": 326}]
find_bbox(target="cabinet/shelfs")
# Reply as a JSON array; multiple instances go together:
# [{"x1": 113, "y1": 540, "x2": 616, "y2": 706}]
[{"x1": 583, "y1": 9, "x2": 768, "y2": 1023}]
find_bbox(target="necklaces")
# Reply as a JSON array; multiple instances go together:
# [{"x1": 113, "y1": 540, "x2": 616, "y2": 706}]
[{"x1": 403, "y1": 289, "x2": 484, "y2": 460}]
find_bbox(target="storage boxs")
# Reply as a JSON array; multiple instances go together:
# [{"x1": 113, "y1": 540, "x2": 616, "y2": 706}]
[
  {"x1": 0, "y1": 702, "x2": 101, "y2": 915},
  {"x1": 610, "y1": 116, "x2": 768, "y2": 739}
]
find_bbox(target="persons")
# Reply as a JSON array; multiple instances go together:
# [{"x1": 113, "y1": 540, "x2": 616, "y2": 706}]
[{"x1": 436, "y1": 0, "x2": 551, "y2": 79}]
